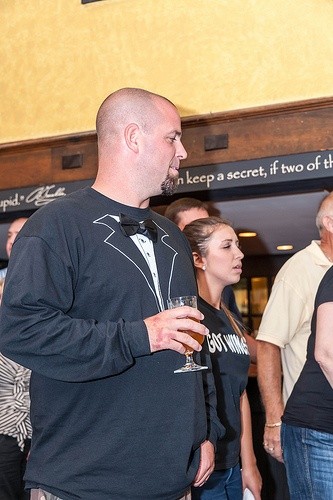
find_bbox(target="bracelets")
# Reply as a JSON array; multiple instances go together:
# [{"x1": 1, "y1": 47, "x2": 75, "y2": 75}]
[{"x1": 265, "y1": 421, "x2": 283, "y2": 427}]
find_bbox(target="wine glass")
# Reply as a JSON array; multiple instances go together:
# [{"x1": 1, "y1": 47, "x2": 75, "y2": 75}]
[{"x1": 167, "y1": 296, "x2": 209, "y2": 373}]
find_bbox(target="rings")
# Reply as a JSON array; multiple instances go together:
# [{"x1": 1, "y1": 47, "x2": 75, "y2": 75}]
[
  {"x1": 263, "y1": 442, "x2": 269, "y2": 446},
  {"x1": 268, "y1": 448, "x2": 274, "y2": 451}
]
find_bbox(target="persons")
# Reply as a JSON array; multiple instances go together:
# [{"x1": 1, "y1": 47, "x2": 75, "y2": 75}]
[
  {"x1": 164, "y1": 198, "x2": 257, "y2": 364},
  {"x1": 0, "y1": 88, "x2": 226, "y2": 500},
  {"x1": 0, "y1": 217, "x2": 32, "y2": 500},
  {"x1": 279, "y1": 265, "x2": 333, "y2": 500},
  {"x1": 255, "y1": 191, "x2": 333, "y2": 500},
  {"x1": 182, "y1": 216, "x2": 262, "y2": 500}
]
